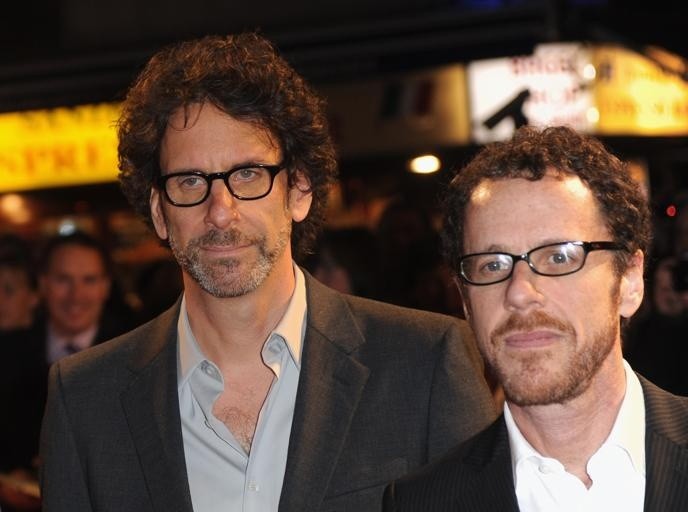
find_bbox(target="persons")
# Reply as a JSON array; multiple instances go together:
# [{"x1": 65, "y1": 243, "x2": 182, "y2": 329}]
[
  {"x1": 382, "y1": 125, "x2": 688, "y2": 512},
  {"x1": 653, "y1": 259, "x2": 688, "y2": 317},
  {"x1": 0, "y1": 228, "x2": 129, "y2": 512},
  {"x1": 39, "y1": 31, "x2": 499, "y2": 512}
]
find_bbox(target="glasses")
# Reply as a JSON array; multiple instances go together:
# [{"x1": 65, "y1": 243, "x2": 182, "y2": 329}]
[
  {"x1": 155, "y1": 157, "x2": 290, "y2": 208},
  {"x1": 456, "y1": 240, "x2": 631, "y2": 287}
]
